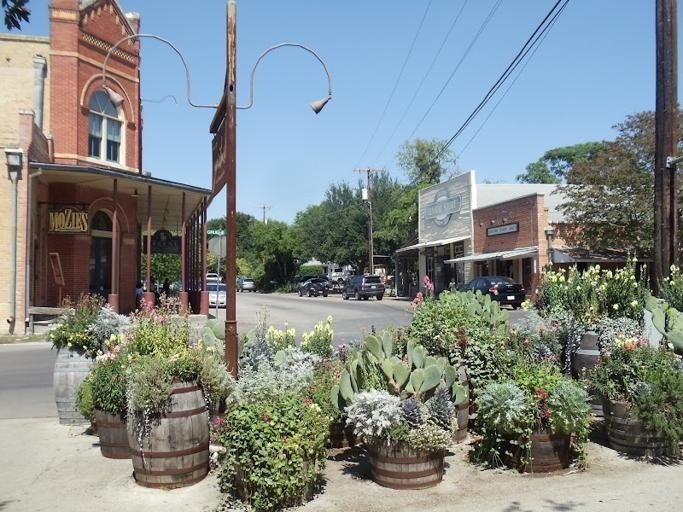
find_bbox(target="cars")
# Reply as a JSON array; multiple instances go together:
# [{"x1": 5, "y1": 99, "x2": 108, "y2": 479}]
[
  {"x1": 296, "y1": 275, "x2": 385, "y2": 301},
  {"x1": 206, "y1": 273, "x2": 226, "y2": 307},
  {"x1": 236, "y1": 278, "x2": 256, "y2": 292},
  {"x1": 459, "y1": 276, "x2": 526, "y2": 309}
]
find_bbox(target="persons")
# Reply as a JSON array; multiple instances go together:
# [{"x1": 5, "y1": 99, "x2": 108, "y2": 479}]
[
  {"x1": 136, "y1": 275, "x2": 170, "y2": 310},
  {"x1": 448, "y1": 278, "x2": 459, "y2": 291},
  {"x1": 99, "y1": 284, "x2": 104, "y2": 294}
]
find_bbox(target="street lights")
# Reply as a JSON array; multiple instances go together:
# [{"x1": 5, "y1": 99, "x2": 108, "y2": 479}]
[{"x1": 101, "y1": 0, "x2": 333, "y2": 383}]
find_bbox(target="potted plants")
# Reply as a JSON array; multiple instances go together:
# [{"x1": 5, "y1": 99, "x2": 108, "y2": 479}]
[
  {"x1": 47, "y1": 291, "x2": 224, "y2": 512},
  {"x1": 469, "y1": 254, "x2": 681, "y2": 476},
  {"x1": 224, "y1": 277, "x2": 468, "y2": 510}
]
